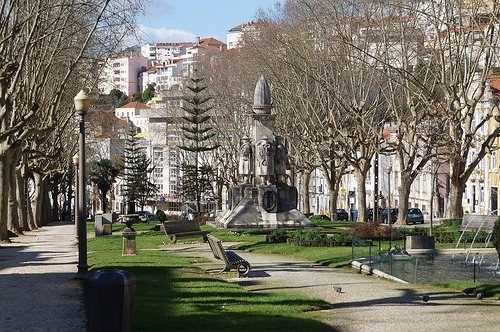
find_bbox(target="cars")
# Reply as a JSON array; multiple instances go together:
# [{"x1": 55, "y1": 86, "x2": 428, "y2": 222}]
[
  {"x1": 135, "y1": 210, "x2": 154, "y2": 220},
  {"x1": 204, "y1": 209, "x2": 222, "y2": 218},
  {"x1": 352, "y1": 205, "x2": 424, "y2": 226},
  {"x1": 325, "y1": 208, "x2": 348, "y2": 222}
]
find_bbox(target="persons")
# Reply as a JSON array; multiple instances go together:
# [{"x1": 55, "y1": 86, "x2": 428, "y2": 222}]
[
  {"x1": 238, "y1": 133, "x2": 254, "y2": 183},
  {"x1": 350, "y1": 204, "x2": 354, "y2": 220},
  {"x1": 275, "y1": 136, "x2": 290, "y2": 183},
  {"x1": 256, "y1": 135, "x2": 274, "y2": 186}
]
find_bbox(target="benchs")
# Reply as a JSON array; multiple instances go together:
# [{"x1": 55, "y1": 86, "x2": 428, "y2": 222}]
[
  {"x1": 160, "y1": 218, "x2": 210, "y2": 244},
  {"x1": 206, "y1": 233, "x2": 251, "y2": 279}
]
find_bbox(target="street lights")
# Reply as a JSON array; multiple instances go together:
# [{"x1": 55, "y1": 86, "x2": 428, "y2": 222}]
[
  {"x1": 72, "y1": 152, "x2": 80, "y2": 245},
  {"x1": 73, "y1": 89, "x2": 94, "y2": 281},
  {"x1": 385, "y1": 164, "x2": 393, "y2": 251}
]
